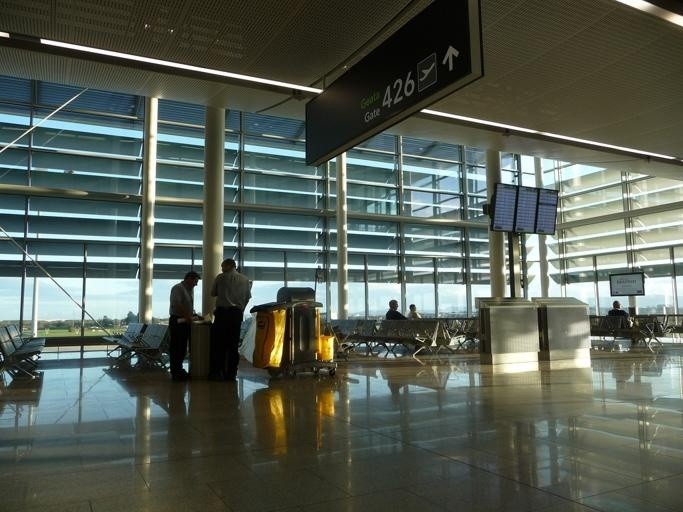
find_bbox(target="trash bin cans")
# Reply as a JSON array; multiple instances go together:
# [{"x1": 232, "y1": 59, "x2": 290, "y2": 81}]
[{"x1": 190, "y1": 320, "x2": 212, "y2": 379}]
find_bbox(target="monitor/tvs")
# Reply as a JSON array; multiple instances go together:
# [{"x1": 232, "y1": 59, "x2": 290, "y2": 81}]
[
  {"x1": 490, "y1": 182, "x2": 559, "y2": 236},
  {"x1": 609, "y1": 271, "x2": 645, "y2": 297}
]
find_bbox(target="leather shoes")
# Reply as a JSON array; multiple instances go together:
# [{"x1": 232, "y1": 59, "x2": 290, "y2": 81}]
[
  {"x1": 172, "y1": 372, "x2": 193, "y2": 380},
  {"x1": 206, "y1": 376, "x2": 236, "y2": 382}
]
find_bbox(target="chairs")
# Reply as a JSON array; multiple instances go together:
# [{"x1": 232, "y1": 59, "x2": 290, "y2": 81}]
[
  {"x1": 1, "y1": 322, "x2": 47, "y2": 380},
  {"x1": 101, "y1": 322, "x2": 171, "y2": 373},
  {"x1": 330, "y1": 317, "x2": 482, "y2": 360},
  {"x1": 590, "y1": 313, "x2": 682, "y2": 353}
]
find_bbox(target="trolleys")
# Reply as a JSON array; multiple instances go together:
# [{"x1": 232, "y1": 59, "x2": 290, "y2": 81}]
[{"x1": 250, "y1": 286, "x2": 340, "y2": 379}]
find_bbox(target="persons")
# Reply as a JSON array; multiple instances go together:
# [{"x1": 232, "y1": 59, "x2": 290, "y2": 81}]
[
  {"x1": 207, "y1": 257, "x2": 252, "y2": 381},
  {"x1": 408, "y1": 304, "x2": 422, "y2": 318},
  {"x1": 385, "y1": 300, "x2": 433, "y2": 354},
  {"x1": 168, "y1": 271, "x2": 201, "y2": 380},
  {"x1": 607, "y1": 300, "x2": 643, "y2": 344}
]
